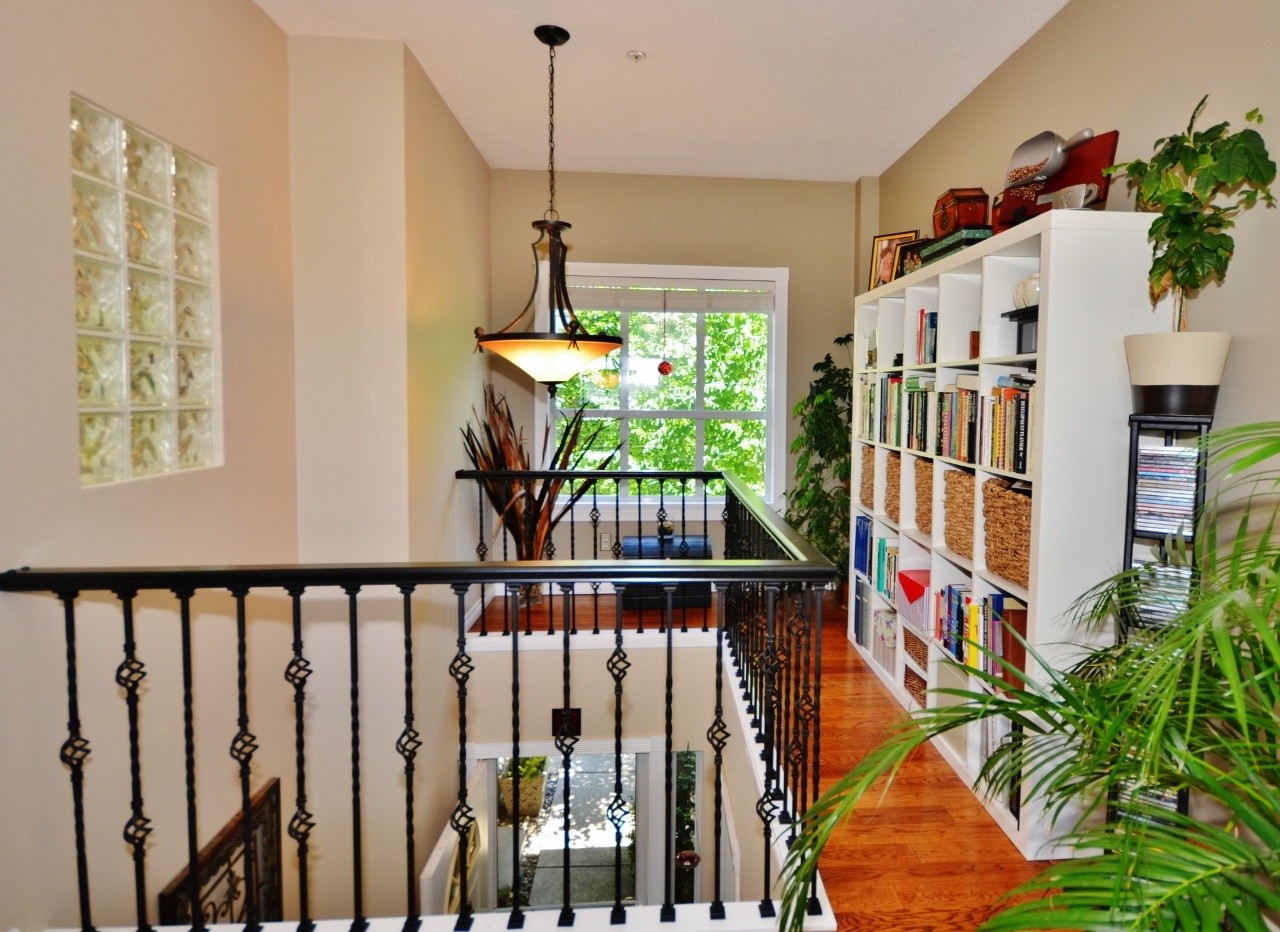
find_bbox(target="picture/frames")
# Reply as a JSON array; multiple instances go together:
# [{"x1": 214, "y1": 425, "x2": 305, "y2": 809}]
[
  {"x1": 157, "y1": 777, "x2": 285, "y2": 927},
  {"x1": 868, "y1": 230, "x2": 921, "y2": 291},
  {"x1": 891, "y1": 238, "x2": 930, "y2": 282}
]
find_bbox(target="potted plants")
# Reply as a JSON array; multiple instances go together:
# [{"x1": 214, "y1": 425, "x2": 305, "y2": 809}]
[
  {"x1": 458, "y1": 384, "x2": 625, "y2": 607},
  {"x1": 498, "y1": 755, "x2": 549, "y2": 817},
  {"x1": 784, "y1": 333, "x2": 855, "y2": 612},
  {"x1": 1104, "y1": 95, "x2": 1277, "y2": 415}
]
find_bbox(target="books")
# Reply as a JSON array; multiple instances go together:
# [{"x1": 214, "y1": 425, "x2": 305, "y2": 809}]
[
  {"x1": 854, "y1": 304, "x2": 1043, "y2": 478},
  {"x1": 844, "y1": 515, "x2": 1029, "y2": 826},
  {"x1": 1109, "y1": 444, "x2": 1205, "y2": 897}
]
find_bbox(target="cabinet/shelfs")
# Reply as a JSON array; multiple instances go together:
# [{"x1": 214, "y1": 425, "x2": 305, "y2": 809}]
[
  {"x1": 848, "y1": 208, "x2": 1173, "y2": 859},
  {"x1": 1104, "y1": 415, "x2": 1211, "y2": 863}
]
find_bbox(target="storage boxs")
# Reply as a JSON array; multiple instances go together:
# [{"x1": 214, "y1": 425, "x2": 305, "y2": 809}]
[
  {"x1": 933, "y1": 188, "x2": 989, "y2": 237},
  {"x1": 919, "y1": 226, "x2": 992, "y2": 268}
]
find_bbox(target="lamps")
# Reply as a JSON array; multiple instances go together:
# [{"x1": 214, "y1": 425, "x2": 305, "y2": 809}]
[{"x1": 481, "y1": 24, "x2": 623, "y2": 398}]
